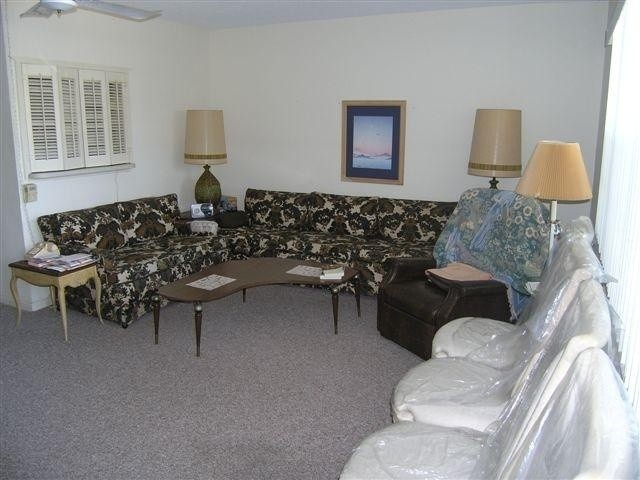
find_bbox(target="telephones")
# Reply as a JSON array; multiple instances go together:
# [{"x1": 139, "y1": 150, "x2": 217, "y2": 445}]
[{"x1": 24, "y1": 242, "x2": 60, "y2": 259}]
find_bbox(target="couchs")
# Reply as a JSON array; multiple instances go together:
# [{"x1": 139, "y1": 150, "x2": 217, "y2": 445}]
[
  {"x1": 209, "y1": 189, "x2": 461, "y2": 296},
  {"x1": 37, "y1": 193, "x2": 232, "y2": 329}
]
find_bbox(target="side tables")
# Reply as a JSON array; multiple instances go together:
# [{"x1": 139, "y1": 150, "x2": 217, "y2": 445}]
[{"x1": 9, "y1": 258, "x2": 104, "y2": 341}]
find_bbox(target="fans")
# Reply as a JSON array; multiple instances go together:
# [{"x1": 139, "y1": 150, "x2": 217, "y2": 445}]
[{"x1": 20, "y1": 0, "x2": 162, "y2": 21}]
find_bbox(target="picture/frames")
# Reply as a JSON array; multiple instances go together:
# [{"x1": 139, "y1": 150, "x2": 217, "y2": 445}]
[{"x1": 340, "y1": 100, "x2": 405, "y2": 185}]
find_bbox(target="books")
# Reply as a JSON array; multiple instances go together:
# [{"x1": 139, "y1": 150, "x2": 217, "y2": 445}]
[
  {"x1": 25, "y1": 253, "x2": 98, "y2": 273},
  {"x1": 323, "y1": 266, "x2": 345, "y2": 277},
  {"x1": 319, "y1": 273, "x2": 344, "y2": 281}
]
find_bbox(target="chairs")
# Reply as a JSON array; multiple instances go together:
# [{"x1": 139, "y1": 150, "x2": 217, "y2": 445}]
[
  {"x1": 376, "y1": 188, "x2": 556, "y2": 362},
  {"x1": 391, "y1": 280, "x2": 612, "y2": 432},
  {"x1": 338, "y1": 347, "x2": 638, "y2": 479},
  {"x1": 430, "y1": 230, "x2": 605, "y2": 371}
]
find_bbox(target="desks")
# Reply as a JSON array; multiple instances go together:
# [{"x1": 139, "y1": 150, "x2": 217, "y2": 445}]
[{"x1": 178, "y1": 209, "x2": 245, "y2": 233}]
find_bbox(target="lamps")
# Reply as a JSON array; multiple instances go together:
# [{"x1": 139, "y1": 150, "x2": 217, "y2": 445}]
[
  {"x1": 465, "y1": 108, "x2": 522, "y2": 188},
  {"x1": 515, "y1": 139, "x2": 592, "y2": 265},
  {"x1": 184, "y1": 108, "x2": 227, "y2": 207}
]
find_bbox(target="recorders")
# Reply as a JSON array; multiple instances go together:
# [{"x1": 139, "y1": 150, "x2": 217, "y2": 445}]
[{"x1": 190, "y1": 203, "x2": 213, "y2": 218}]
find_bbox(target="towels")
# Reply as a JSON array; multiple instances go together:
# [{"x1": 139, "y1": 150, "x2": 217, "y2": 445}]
[{"x1": 425, "y1": 260, "x2": 493, "y2": 283}]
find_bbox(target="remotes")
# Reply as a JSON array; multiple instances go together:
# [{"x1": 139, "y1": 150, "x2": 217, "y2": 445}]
[{"x1": 46, "y1": 265, "x2": 66, "y2": 273}]
